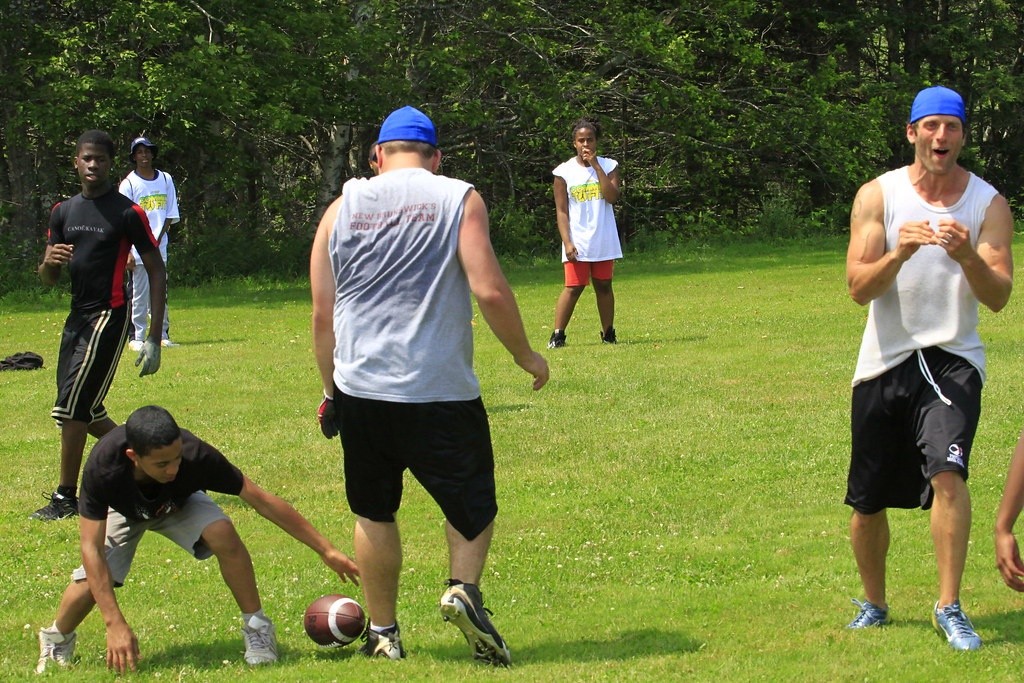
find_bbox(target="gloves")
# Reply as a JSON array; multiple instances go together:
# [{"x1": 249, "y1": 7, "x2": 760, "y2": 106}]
[{"x1": 134, "y1": 336, "x2": 161, "y2": 377}]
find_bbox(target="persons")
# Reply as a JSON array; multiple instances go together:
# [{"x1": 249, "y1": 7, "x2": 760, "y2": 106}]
[
  {"x1": 37, "y1": 406, "x2": 361, "y2": 676},
  {"x1": 995, "y1": 431, "x2": 1024, "y2": 592},
  {"x1": 842, "y1": 87, "x2": 1014, "y2": 653},
  {"x1": 119, "y1": 138, "x2": 181, "y2": 350},
  {"x1": 547, "y1": 123, "x2": 623, "y2": 348},
  {"x1": 28, "y1": 133, "x2": 167, "y2": 521},
  {"x1": 310, "y1": 108, "x2": 549, "y2": 669}
]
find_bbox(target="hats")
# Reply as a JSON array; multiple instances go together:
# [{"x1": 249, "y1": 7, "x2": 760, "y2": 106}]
[
  {"x1": 910, "y1": 86, "x2": 967, "y2": 129},
  {"x1": 128, "y1": 137, "x2": 158, "y2": 163},
  {"x1": 377, "y1": 105, "x2": 436, "y2": 149}
]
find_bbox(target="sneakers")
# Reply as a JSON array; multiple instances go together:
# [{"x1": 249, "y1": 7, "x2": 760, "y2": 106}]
[
  {"x1": 843, "y1": 598, "x2": 893, "y2": 629},
  {"x1": 34, "y1": 623, "x2": 77, "y2": 674},
  {"x1": 600, "y1": 326, "x2": 617, "y2": 345},
  {"x1": 128, "y1": 339, "x2": 145, "y2": 351},
  {"x1": 438, "y1": 577, "x2": 511, "y2": 667},
  {"x1": 546, "y1": 329, "x2": 566, "y2": 350},
  {"x1": 26, "y1": 490, "x2": 79, "y2": 521},
  {"x1": 357, "y1": 618, "x2": 407, "y2": 659},
  {"x1": 160, "y1": 339, "x2": 180, "y2": 347},
  {"x1": 240, "y1": 614, "x2": 278, "y2": 667},
  {"x1": 931, "y1": 599, "x2": 981, "y2": 653}
]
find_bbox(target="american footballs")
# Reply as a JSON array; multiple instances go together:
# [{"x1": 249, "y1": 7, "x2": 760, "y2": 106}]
[{"x1": 305, "y1": 595, "x2": 367, "y2": 647}]
